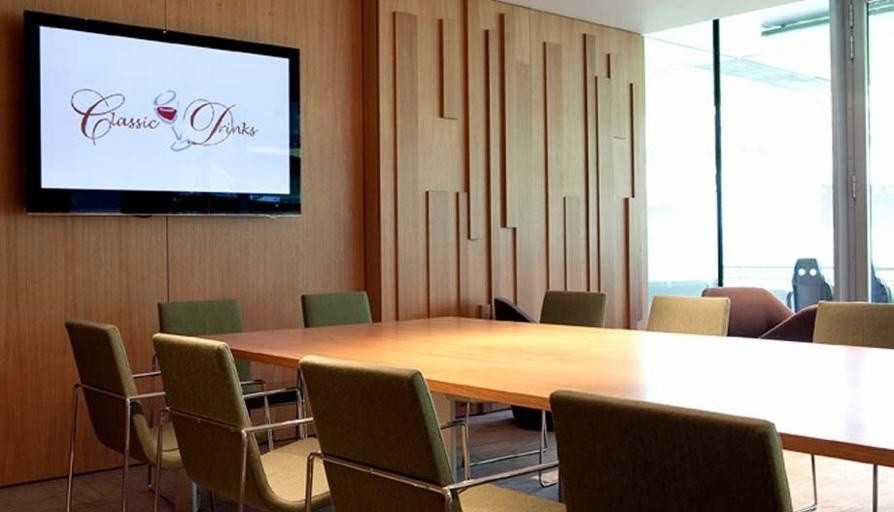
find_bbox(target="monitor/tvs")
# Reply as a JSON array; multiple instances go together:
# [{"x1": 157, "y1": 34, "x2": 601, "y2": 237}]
[{"x1": 22, "y1": 9, "x2": 301, "y2": 218}]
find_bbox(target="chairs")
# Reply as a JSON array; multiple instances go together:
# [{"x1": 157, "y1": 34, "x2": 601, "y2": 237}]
[
  {"x1": 787, "y1": 256, "x2": 891, "y2": 308},
  {"x1": 65, "y1": 289, "x2": 893, "y2": 511}
]
